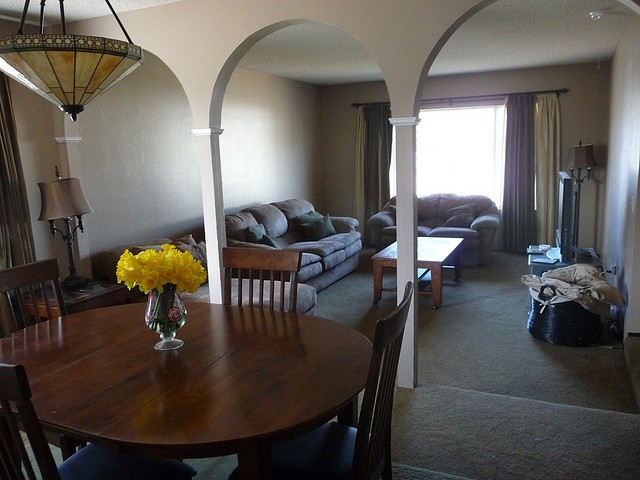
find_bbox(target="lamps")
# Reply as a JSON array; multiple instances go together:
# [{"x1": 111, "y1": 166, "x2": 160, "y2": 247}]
[
  {"x1": 38, "y1": 165, "x2": 89, "y2": 289},
  {"x1": 0, "y1": 0, "x2": 143, "y2": 121},
  {"x1": 570, "y1": 142, "x2": 597, "y2": 246}
]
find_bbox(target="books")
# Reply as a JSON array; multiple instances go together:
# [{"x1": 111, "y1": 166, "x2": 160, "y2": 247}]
[{"x1": 531, "y1": 258, "x2": 558, "y2": 264}]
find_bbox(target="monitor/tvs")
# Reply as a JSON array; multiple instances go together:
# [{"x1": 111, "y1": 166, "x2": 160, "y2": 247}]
[{"x1": 546, "y1": 171, "x2": 577, "y2": 266}]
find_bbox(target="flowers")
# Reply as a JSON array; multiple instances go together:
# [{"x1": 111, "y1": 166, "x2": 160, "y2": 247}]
[{"x1": 117, "y1": 244, "x2": 207, "y2": 333}]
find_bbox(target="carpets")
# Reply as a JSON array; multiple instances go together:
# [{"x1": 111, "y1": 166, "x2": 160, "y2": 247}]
[
  {"x1": 392, "y1": 381, "x2": 640, "y2": 480},
  {"x1": 185, "y1": 450, "x2": 470, "y2": 480}
]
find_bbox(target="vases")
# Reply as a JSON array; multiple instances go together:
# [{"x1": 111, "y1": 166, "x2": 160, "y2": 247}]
[{"x1": 146, "y1": 293, "x2": 186, "y2": 349}]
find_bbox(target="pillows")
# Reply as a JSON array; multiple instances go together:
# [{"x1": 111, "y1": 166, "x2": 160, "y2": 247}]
[
  {"x1": 445, "y1": 204, "x2": 476, "y2": 227},
  {"x1": 178, "y1": 241, "x2": 208, "y2": 284},
  {"x1": 389, "y1": 204, "x2": 396, "y2": 219},
  {"x1": 129, "y1": 235, "x2": 196, "y2": 255},
  {"x1": 246, "y1": 224, "x2": 266, "y2": 243},
  {"x1": 293, "y1": 210, "x2": 323, "y2": 231},
  {"x1": 301, "y1": 213, "x2": 336, "y2": 242},
  {"x1": 103, "y1": 238, "x2": 171, "y2": 279},
  {"x1": 261, "y1": 234, "x2": 280, "y2": 249}
]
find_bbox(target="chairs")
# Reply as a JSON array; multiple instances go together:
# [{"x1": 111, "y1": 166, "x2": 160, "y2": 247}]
[
  {"x1": 1, "y1": 364, "x2": 196, "y2": 479},
  {"x1": 0, "y1": 259, "x2": 88, "y2": 462},
  {"x1": 229, "y1": 281, "x2": 414, "y2": 480},
  {"x1": 222, "y1": 248, "x2": 302, "y2": 312}
]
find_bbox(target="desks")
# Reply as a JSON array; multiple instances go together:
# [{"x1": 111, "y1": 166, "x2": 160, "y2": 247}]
[
  {"x1": 530, "y1": 246, "x2": 603, "y2": 308},
  {"x1": 372, "y1": 236, "x2": 464, "y2": 308},
  {"x1": 8, "y1": 277, "x2": 138, "y2": 322}
]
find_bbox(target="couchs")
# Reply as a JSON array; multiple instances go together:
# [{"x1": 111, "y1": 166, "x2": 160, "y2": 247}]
[
  {"x1": 96, "y1": 235, "x2": 318, "y2": 318},
  {"x1": 226, "y1": 198, "x2": 362, "y2": 291},
  {"x1": 367, "y1": 193, "x2": 500, "y2": 266}
]
